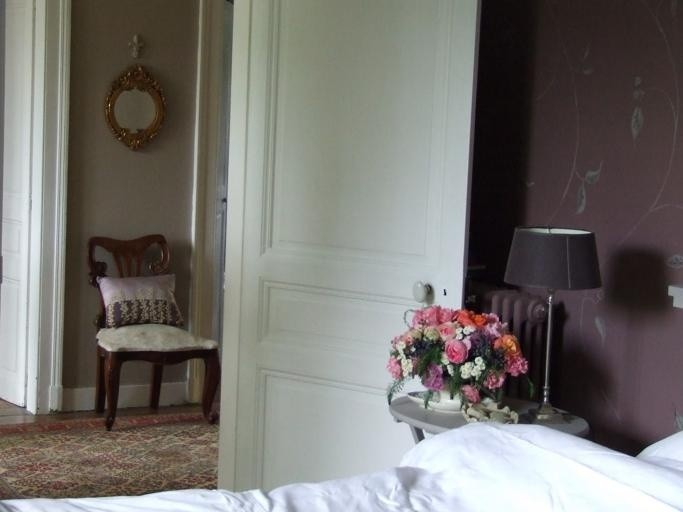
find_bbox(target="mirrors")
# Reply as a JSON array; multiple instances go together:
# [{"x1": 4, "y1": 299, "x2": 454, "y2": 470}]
[{"x1": 101, "y1": 64, "x2": 166, "y2": 152}]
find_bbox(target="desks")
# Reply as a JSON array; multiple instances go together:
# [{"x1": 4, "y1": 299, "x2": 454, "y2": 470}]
[{"x1": 386, "y1": 395, "x2": 589, "y2": 449}]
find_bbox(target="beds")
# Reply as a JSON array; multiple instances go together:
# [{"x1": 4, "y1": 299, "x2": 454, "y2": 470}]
[{"x1": 0, "y1": 417, "x2": 683, "y2": 508}]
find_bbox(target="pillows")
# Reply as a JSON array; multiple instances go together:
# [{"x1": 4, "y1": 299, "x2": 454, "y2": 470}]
[{"x1": 90, "y1": 271, "x2": 185, "y2": 332}]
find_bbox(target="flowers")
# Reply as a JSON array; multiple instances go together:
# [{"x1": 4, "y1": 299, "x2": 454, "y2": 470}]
[{"x1": 388, "y1": 305, "x2": 534, "y2": 419}]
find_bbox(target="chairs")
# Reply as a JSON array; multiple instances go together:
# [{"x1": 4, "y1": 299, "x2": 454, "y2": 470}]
[{"x1": 85, "y1": 233, "x2": 219, "y2": 428}]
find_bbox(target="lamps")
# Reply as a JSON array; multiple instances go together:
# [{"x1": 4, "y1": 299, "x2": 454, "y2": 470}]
[{"x1": 500, "y1": 227, "x2": 602, "y2": 422}]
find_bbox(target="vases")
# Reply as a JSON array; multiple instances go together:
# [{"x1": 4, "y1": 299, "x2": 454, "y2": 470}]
[{"x1": 435, "y1": 386, "x2": 494, "y2": 402}]
